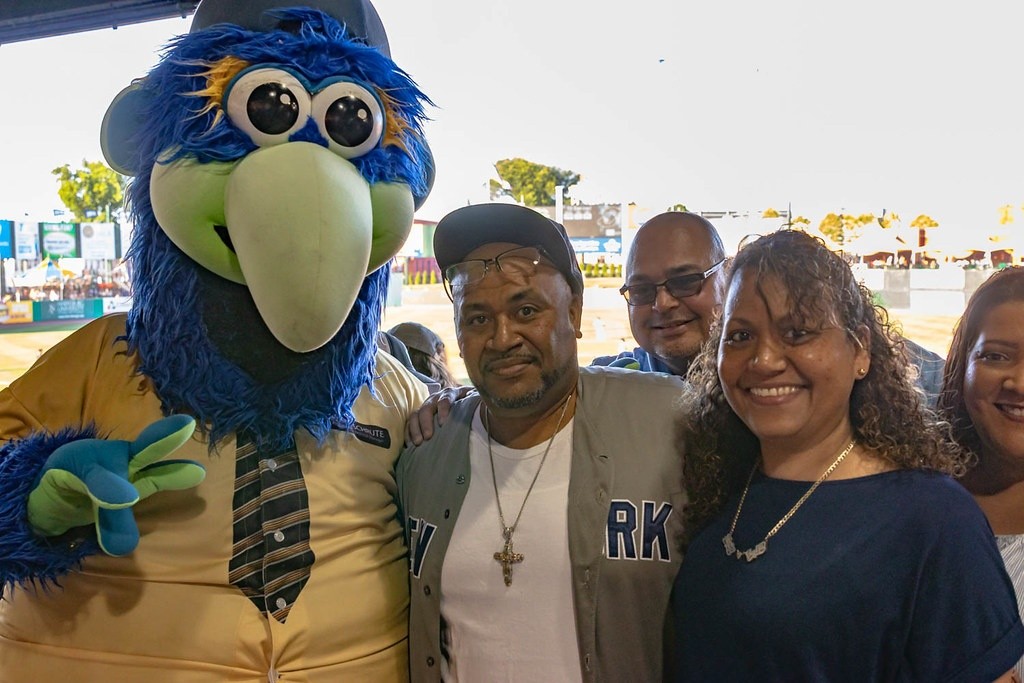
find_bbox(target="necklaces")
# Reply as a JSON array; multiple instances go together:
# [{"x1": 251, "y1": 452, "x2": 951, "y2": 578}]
[
  {"x1": 722, "y1": 434, "x2": 858, "y2": 562},
  {"x1": 486, "y1": 395, "x2": 571, "y2": 587}
]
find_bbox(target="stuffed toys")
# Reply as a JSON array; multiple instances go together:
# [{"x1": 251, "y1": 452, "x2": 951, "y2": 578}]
[{"x1": 0, "y1": 0, "x2": 431, "y2": 683}]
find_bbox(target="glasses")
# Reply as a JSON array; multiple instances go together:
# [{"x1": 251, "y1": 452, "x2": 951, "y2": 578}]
[
  {"x1": 619, "y1": 258, "x2": 728, "y2": 306},
  {"x1": 442, "y1": 242, "x2": 557, "y2": 303}
]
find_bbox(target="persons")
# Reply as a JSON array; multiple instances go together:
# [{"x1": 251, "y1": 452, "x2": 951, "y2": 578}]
[
  {"x1": 396, "y1": 203, "x2": 701, "y2": 683},
  {"x1": 935, "y1": 264, "x2": 1024, "y2": 683},
  {"x1": 663, "y1": 231, "x2": 1024, "y2": 683},
  {"x1": 403, "y1": 203, "x2": 733, "y2": 447},
  {"x1": 376, "y1": 319, "x2": 462, "y2": 396},
  {"x1": 1, "y1": 270, "x2": 132, "y2": 302}
]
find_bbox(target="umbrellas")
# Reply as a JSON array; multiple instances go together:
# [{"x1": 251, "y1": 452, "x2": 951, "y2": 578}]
[{"x1": 16, "y1": 254, "x2": 76, "y2": 286}]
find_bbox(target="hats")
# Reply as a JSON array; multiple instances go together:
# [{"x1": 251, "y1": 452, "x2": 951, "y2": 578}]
[
  {"x1": 433, "y1": 203, "x2": 584, "y2": 295},
  {"x1": 388, "y1": 322, "x2": 443, "y2": 364}
]
